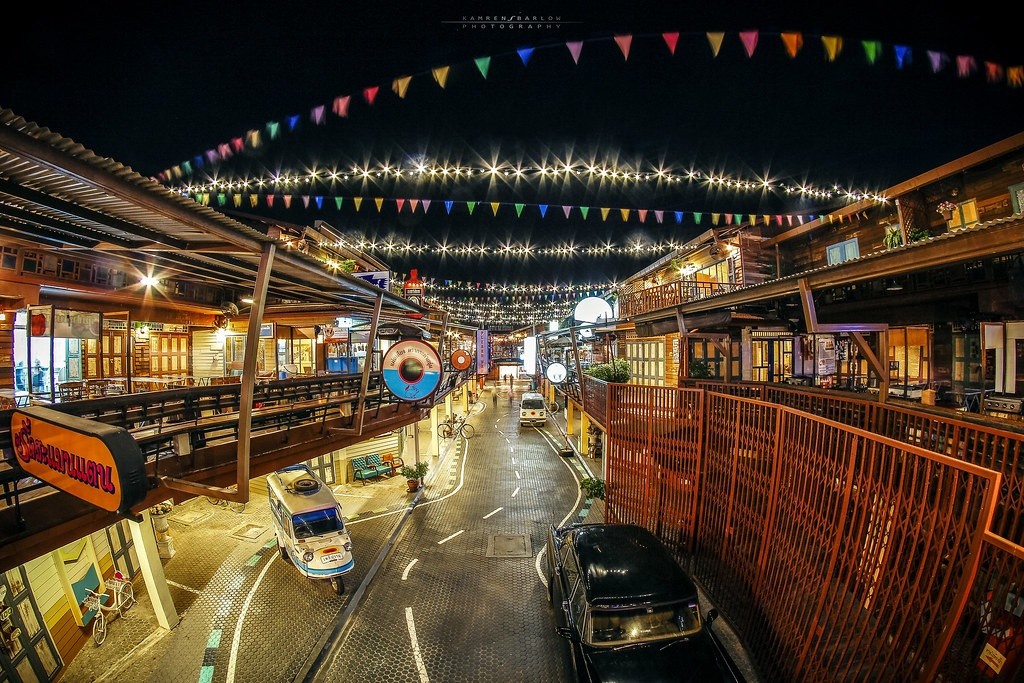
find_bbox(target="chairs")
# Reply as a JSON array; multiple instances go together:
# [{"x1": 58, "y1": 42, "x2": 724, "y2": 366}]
[
  {"x1": 86, "y1": 380, "x2": 111, "y2": 400},
  {"x1": 59, "y1": 381, "x2": 84, "y2": 403},
  {"x1": 383, "y1": 453, "x2": 404, "y2": 477},
  {"x1": 366, "y1": 454, "x2": 392, "y2": 482},
  {"x1": 352, "y1": 456, "x2": 377, "y2": 486}
]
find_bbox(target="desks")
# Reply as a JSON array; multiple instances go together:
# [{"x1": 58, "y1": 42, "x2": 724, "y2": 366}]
[
  {"x1": 946, "y1": 386, "x2": 995, "y2": 414},
  {"x1": 833, "y1": 375, "x2": 867, "y2": 384},
  {"x1": 0, "y1": 388, "x2": 29, "y2": 408},
  {"x1": 890, "y1": 381, "x2": 942, "y2": 402},
  {"x1": 166, "y1": 375, "x2": 220, "y2": 416},
  {"x1": 104, "y1": 377, "x2": 183, "y2": 428}
]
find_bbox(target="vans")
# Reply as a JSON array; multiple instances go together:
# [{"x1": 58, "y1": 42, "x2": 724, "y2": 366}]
[{"x1": 518, "y1": 392, "x2": 549, "y2": 428}]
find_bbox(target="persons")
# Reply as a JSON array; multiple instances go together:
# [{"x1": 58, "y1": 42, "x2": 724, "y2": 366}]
[
  {"x1": 504, "y1": 374, "x2": 506, "y2": 382},
  {"x1": 508, "y1": 387, "x2": 514, "y2": 402},
  {"x1": 499, "y1": 345, "x2": 513, "y2": 357},
  {"x1": 16, "y1": 360, "x2": 44, "y2": 392},
  {"x1": 510, "y1": 374, "x2": 514, "y2": 388},
  {"x1": 492, "y1": 387, "x2": 498, "y2": 404}
]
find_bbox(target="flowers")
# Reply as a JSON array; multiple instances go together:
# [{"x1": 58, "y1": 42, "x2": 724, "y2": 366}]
[
  {"x1": 151, "y1": 503, "x2": 172, "y2": 515},
  {"x1": 935, "y1": 201, "x2": 957, "y2": 212}
]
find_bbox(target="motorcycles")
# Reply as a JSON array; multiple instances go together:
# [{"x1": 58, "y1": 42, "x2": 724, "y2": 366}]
[{"x1": 264, "y1": 463, "x2": 355, "y2": 596}]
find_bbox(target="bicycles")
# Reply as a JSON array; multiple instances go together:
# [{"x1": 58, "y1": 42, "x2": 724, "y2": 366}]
[
  {"x1": 206, "y1": 486, "x2": 246, "y2": 514},
  {"x1": 84, "y1": 577, "x2": 140, "y2": 645},
  {"x1": 437, "y1": 416, "x2": 475, "y2": 439}
]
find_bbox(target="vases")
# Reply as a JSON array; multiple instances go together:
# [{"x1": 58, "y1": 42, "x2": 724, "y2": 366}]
[
  {"x1": 150, "y1": 513, "x2": 170, "y2": 533},
  {"x1": 943, "y1": 210, "x2": 952, "y2": 221}
]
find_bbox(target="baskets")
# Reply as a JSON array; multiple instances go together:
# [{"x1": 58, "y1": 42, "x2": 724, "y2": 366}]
[
  {"x1": 82, "y1": 594, "x2": 101, "y2": 610},
  {"x1": 106, "y1": 578, "x2": 122, "y2": 590}
]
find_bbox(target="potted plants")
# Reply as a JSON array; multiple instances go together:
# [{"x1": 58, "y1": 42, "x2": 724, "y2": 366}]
[
  {"x1": 583, "y1": 356, "x2": 631, "y2": 410},
  {"x1": 399, "y1": 461, "x2": 430, "y2": 493}
]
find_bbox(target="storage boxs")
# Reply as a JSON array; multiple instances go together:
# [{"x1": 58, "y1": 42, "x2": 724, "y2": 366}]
[{"x1": 327, "y1": 356, "x2": 358, "y2": 374}]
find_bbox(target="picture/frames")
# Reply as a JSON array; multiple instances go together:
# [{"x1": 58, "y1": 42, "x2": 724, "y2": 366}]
[{"x1": 259, "y1": 323, "x2": 273, "y2": 339}]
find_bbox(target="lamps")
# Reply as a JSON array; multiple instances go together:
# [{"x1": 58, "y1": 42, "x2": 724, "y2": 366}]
[{"x1": 132, "y1": 321, "x2": 152, "y2": 340}]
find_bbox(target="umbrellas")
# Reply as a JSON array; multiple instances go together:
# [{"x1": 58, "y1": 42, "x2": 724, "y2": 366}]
[{"x1": 554, "y1": 337, "x2": 582, "y2": 352}]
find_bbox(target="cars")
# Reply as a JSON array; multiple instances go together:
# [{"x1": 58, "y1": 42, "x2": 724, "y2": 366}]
[{"x1": 545, "y1": 520, "x2": 728, "y2": 683}]
